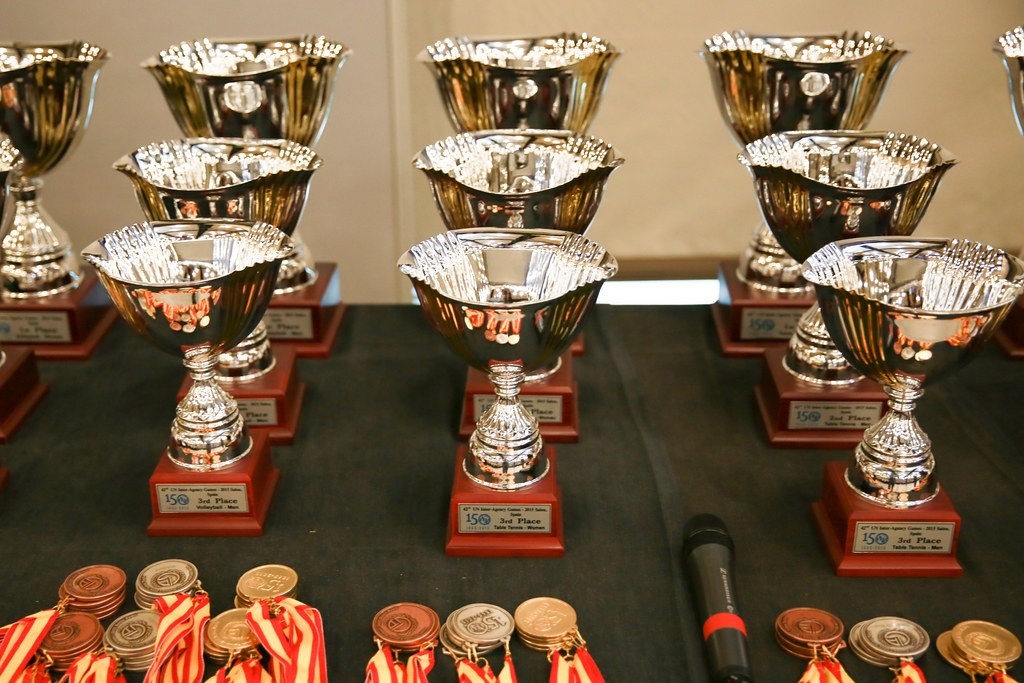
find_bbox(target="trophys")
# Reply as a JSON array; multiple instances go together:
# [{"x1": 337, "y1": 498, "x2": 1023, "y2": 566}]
[{"x1": 2, "y1": 28, "x2": 1023, "y2": 582}]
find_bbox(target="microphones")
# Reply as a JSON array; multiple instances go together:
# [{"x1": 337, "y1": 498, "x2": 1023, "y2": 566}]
[{"x1": 681, "y1": 513, "x2": 752, "y2": 683}]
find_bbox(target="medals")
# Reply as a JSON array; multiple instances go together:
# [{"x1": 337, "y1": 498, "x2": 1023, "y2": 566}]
[
  {"x1": 769, "y1": 604, "x2": 1024, "y2": 683},
  {"x1": 35, "y1": 554, "x2": 583, "y2": 681}
]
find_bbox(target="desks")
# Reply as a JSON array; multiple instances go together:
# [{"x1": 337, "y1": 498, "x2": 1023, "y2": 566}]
[{"x1": 0, "y1": 303, "x2": 1021, "y2": 683}]
